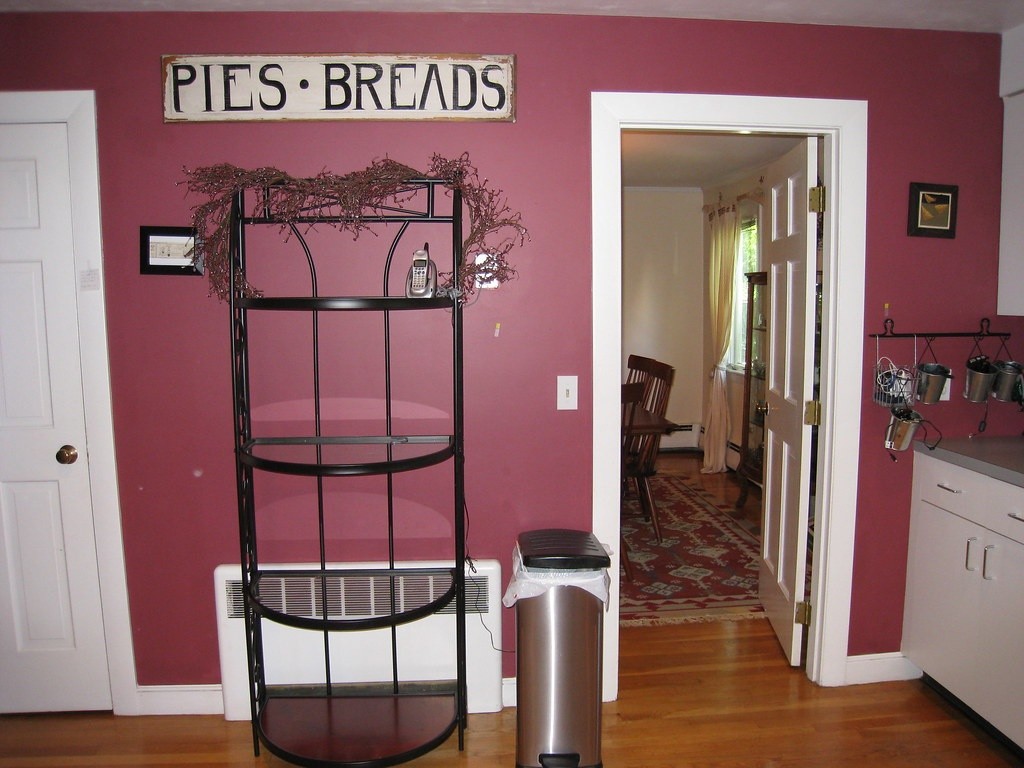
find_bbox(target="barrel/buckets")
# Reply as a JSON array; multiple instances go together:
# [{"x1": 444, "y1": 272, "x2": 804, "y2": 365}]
[
  {"x1": 915, "y1": 363, "x2": 950, "y2": 405},
  {"x1": 885, "y1": 406, "x2": 924, "y2": 452},
  {"x1": 963, "y1": 362, "x2": 999, "y2": 404},
  {"x1": 990, "y1": 361, "x2": 1024, "y2": 403}
]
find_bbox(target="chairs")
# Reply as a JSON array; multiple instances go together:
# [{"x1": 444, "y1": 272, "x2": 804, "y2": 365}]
[{"x1": 621, "y1": 354, "x2": 676, "y2": 543}]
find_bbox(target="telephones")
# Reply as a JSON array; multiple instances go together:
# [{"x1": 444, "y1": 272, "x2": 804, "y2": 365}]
[{"x1": 405, "y1": 242, "x2": 437, "y2": 298}]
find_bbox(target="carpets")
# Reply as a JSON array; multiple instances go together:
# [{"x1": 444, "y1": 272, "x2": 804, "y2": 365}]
[{"x1": 618, "y1": 471, "x2": 813, "y2": 635}]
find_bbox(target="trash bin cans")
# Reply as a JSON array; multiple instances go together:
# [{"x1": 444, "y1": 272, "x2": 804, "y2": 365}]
[{"x1": 511, "y1": 529, "x2": 611, "y2": 768}]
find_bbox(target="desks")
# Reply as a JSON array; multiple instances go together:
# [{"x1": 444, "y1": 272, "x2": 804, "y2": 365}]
[{"x1": 622, "y1": 404, "x2": 679, "y2": 437}]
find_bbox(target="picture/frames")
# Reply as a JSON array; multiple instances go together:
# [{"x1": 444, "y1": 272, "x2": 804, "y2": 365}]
[
  {"x1": 907, "y1": 182, "x2": 959, "y2": 239},
  {"x1": 139, "y1": 225, "x2": 205, "y2": 276}
]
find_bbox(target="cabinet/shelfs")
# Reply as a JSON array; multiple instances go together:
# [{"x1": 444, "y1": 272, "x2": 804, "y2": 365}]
[
  {"x1": 900, "y1": 450, "x2": 1024, "y2": 768},
  {"x1": 734, "y1": 271, "x2": 823, "y2": 528},
  {"x1": 220, "y1": 170, "x2": 470, "y2": 768}
]
